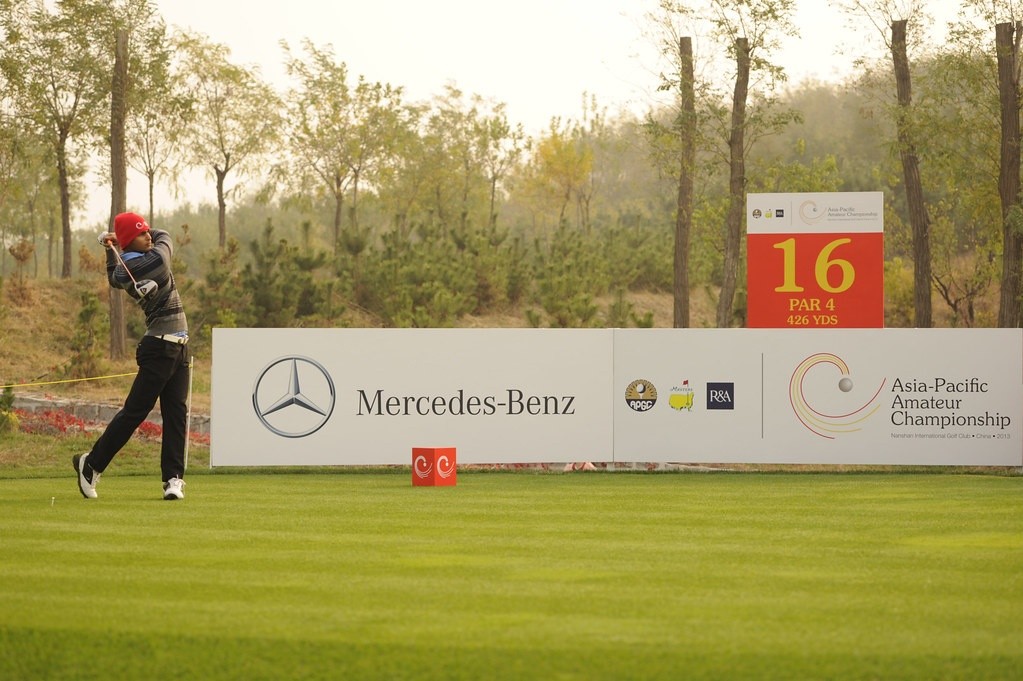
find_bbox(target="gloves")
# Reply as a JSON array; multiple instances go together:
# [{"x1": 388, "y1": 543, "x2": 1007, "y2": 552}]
[{"x1": 97, "y1": 231, "x2": 112, "y2": 250}]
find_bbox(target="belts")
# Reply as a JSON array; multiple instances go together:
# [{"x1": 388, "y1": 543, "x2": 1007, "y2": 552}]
[{"x1": 155, "y1": 333, "x2": 189, "y2": 346}]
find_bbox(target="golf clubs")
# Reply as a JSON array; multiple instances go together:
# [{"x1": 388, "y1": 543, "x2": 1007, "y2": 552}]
[{"x1": 107, "y1": 240, "x2": 158, "y2": 302}]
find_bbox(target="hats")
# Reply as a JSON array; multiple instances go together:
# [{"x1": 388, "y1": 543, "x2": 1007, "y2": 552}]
[{"x1": 114, "y1": 213, "x2": 150, "y2": 250}]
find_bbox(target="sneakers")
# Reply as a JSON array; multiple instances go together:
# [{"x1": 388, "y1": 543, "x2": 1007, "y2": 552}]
[
  {"x1": 73, "y1": 452, "x2": 100, "y2": 498},
  {"x1": 163, "y1": 474, "x2": 186, "y2": 500}
]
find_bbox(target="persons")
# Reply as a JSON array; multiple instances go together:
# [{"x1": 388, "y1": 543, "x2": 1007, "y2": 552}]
[{"x1": 73, "y1": 214, "x2": 191, "y2": 500}]
